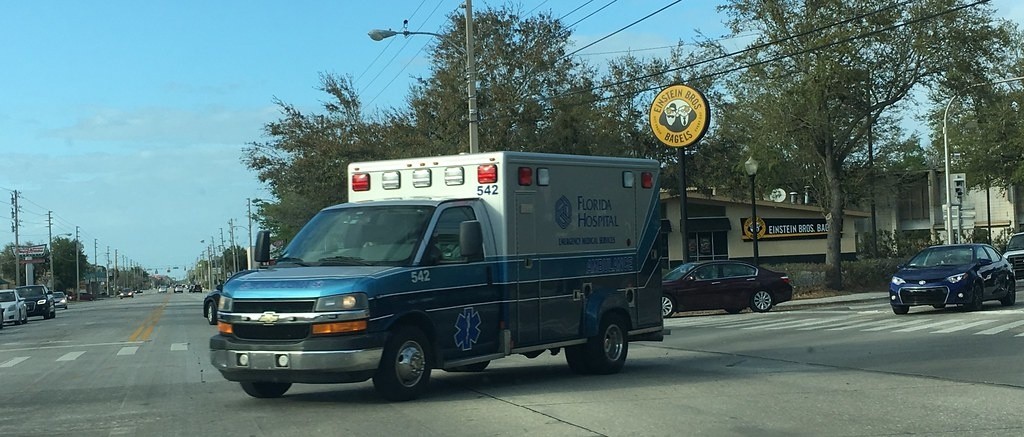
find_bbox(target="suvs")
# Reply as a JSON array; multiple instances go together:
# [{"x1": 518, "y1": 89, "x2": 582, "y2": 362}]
[
  {"x1": 1003, "y1": 231, "x2": 1024, "y2": 280},
  {"x1": 15, "y1": 284, "x2": 56, "y2": 319}
]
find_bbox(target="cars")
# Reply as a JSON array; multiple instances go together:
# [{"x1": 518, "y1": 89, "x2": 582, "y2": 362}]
[
  {"x1": 662, "y1": 260, "x2": 793, "y2": 318},
  {"x1": 53, "y1": 292, "x2": 69, "y2": 309},
  {"x1": 888, "y1": 243, "x2": 1016, "y2": 315},
  {"x1": 0, "y1": 289, "x2": 28, "y2": 325},
  {"x1": 203, "y1": 269, "x2": 264, "y2": 326},
  {"x1": 119, "y1": 283, "x2": 203, "y2": 299},
  {"x1": 69, "y1": 293, "x2": 95, "y2": 301}
]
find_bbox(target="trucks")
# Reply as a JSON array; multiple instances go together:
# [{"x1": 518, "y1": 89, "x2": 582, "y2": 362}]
[{"x1": 208, "y1": 152, "x2": 673, "y2": 403}]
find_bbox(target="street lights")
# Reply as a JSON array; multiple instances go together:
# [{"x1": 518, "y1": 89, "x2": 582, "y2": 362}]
[
  {"x1": 745, "y1": 156, "x2": 760, "y2": 268},
  {"x1": 367, "y1": 1, "x2": 479, "y2": 156},
  {"x1": 200, "y1": 195, "x2": 278, "y2": 290}
]
